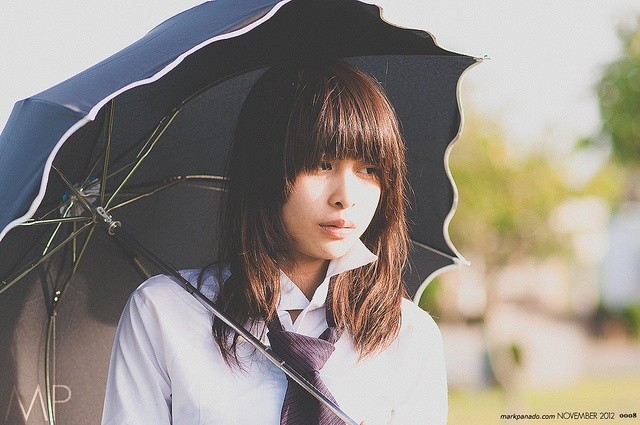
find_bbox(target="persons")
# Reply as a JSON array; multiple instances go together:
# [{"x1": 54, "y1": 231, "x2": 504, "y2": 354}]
[{"x1": 101, "y1": 54, "x2": 449, "y2": 425}]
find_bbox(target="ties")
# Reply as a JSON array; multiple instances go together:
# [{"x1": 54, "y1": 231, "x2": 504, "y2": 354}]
[{"x1": 262, "y1": 303, "x2": 345, "y2": 425}]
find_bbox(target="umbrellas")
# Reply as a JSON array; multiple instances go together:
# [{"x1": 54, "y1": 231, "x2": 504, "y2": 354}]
[{"x1": 0, "y1": 0, "x2": 493, "y2": 424}]
